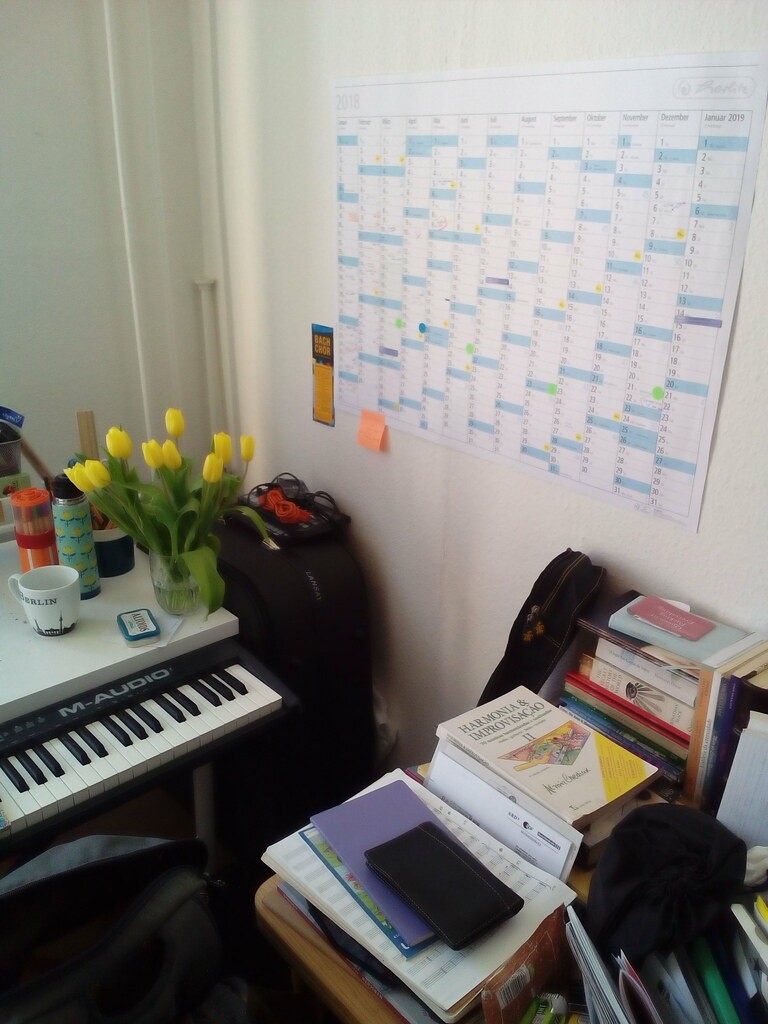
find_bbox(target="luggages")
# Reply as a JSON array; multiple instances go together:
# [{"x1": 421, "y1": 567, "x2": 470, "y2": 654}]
[{"x1": 165, "y1": 499, "x2": 368, "y2": 857}]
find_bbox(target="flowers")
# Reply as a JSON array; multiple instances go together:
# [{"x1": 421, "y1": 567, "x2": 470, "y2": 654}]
[{"x1": 63, "y1": 408, "x2": 256, "y2": 584}]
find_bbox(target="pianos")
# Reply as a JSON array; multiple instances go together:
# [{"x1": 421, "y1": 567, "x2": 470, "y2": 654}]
[{"x1": 0, "y1": 644, "x2": 297, "y2": 848}]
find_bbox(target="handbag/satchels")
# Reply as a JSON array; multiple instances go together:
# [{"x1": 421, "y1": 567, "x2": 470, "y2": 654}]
[{"x1": 0, "y1": 833, "x2": 269, "y2": 1024}]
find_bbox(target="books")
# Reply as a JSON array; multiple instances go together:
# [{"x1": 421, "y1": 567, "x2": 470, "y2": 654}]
[{"x1": 260, "y1": 590, "x2": 768, "y2": 1024}]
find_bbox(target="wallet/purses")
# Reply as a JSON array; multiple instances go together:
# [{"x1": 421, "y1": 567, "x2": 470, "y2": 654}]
[{"x1": 366, "y1": 822, "x2": 524, "y2": 950}]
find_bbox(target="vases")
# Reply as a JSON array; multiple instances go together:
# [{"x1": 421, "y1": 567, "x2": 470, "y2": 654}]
[{"x1": 149, "y1": 546, "x2": 201, "y2": 616}]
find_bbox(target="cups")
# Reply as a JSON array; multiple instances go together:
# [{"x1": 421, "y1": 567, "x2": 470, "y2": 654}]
[
  {"x1": 92, "y1": 526, "x2": 136, "y2": 577},
  {"x1": 11, "y1": 488, "x2": 58, "y2": 572},
  {"x1": 7, "y1": 565, "x2": 81, "y2": 636}
]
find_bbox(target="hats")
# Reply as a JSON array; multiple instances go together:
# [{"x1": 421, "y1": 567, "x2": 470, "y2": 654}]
[{"x1": 586, "y1": 803, "x2": 746, "y2": 960}]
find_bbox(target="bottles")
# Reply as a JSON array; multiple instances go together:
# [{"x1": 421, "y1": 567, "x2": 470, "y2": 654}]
[{"x1": 51, "y1": 473, "x2": 101, "y2": 600}]
